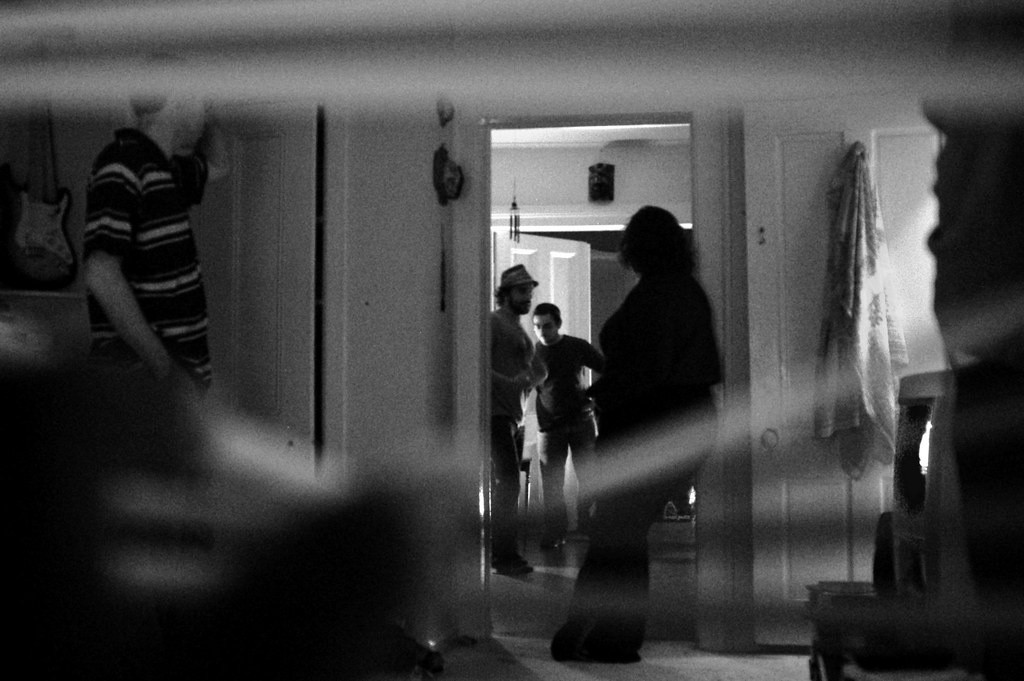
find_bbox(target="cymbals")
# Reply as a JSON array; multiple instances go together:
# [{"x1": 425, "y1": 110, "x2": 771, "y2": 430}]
[{"x1": 0, "y1": 289, "x2": 93, "y2": 376}]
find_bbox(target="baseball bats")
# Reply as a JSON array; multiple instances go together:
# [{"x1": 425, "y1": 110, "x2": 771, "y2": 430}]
[{"x1": 421, "y1": 99, "x2": 457, "y2": 417}]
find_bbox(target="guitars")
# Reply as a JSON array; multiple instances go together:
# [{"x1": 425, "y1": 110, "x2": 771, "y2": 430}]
[{"x1": 10, "y1": 104, "x2": 78, "y2": 294}]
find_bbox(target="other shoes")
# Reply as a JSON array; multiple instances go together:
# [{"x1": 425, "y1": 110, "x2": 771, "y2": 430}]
[
  {"x1": 541, "y1": 532, "x2": 565, "y2": 547},
  {"x1": 491, "y1": 554, "x2": 533, "y2": 575}
]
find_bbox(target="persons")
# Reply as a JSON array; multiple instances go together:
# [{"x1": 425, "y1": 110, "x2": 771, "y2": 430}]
[
  {"x1": 530, "y1": 303, "x2": 603, "y2": 548},
  {"x1": 80, "y1": 50, "x2": 233, "y2": 405},
  {"x1": 554, "y1": 203, "x2": 726, "y2": 663},
  {"x1": 482, "y1": 265, "x2": 549, "y2": 576}
]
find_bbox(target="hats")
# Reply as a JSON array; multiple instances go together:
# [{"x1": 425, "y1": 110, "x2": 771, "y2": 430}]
[{"x1": 501, "y1": 265, "x2": 538, "y2": 289}]
